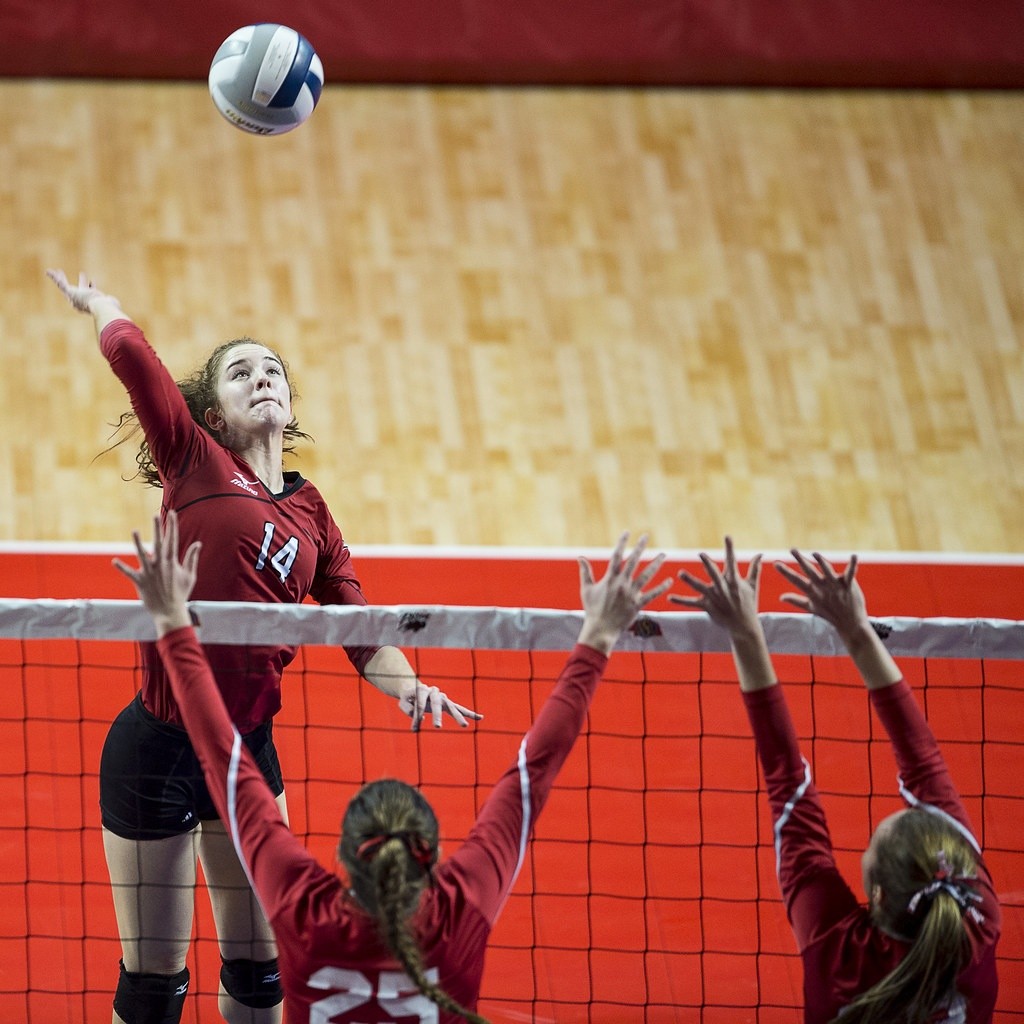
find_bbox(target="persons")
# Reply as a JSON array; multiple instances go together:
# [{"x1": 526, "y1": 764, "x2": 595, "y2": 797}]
[
  {"x1": 46, "y1": 268, "x2": 486, "y2": 1024},
  {"x1": 669, "y1": 535, "x2": 1004, "y2": 1024},
  {"x1": 112, "y1": 511, "x2": 673, "y2": 1024}
]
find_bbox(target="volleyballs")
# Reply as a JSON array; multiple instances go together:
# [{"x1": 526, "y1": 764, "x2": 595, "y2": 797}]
[{"x1": 208, "y1": 23, "x2": 324, "y2": 136}]
